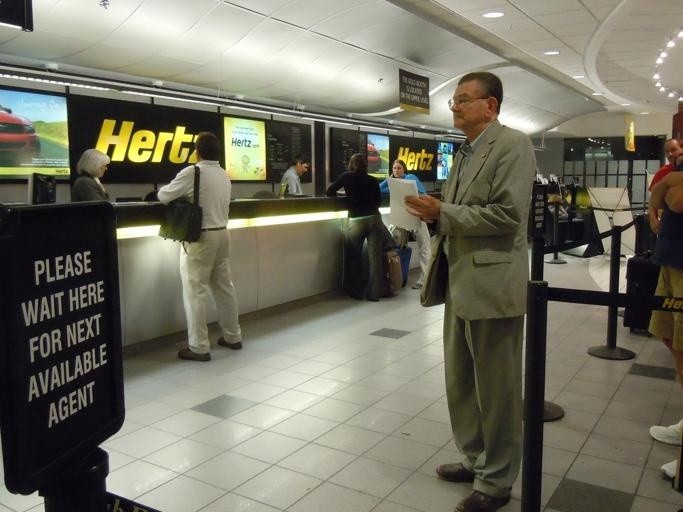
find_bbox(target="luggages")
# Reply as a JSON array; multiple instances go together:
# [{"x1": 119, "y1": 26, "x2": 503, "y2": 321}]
[
  {"x1": 383, "y1": 246, "x2": 412, "y2": 292},
  {"x1": 623, "y1": 250, "x2": 661, "y2": 331}
]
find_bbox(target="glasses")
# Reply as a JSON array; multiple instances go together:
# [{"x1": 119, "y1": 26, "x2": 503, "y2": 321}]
[{"x1": 447, "y1": 97, "x2": 488, "y2": 108}]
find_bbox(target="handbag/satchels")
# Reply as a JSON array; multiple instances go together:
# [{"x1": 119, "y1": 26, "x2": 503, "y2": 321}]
[
  {"x1": 389, "y1": 224, "x2": 408, "y2": 246},
  {"x1": 158, "y1": 199, "x2": 202, "y2": 242}
]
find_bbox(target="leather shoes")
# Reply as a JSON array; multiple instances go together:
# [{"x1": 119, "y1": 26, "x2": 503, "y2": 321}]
[
  {"x1": 455, "y1": 489, "x2": 511, "y2": 512},
  {"x1": 436, "y1": 462, "x2": 474, "y2": 480}
]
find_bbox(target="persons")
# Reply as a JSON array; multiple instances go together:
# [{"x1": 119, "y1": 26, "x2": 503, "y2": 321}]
[
  {"x1": 72, "y1": 149, "x2": 111, "y2": 200},
  {"x1": 280, "y1": 155, "x2": 309, "y2": 194},
  {"x1": 648, "y1": 138, "x2": 679, "y2": 232},
  {"x1": 157, "y1": 132, "x2": 242, "y2": 361},
  {"x1": 379, "y1": 160, "x2": 432, "y2": 289},
  {"x1": 406, "y1": 71, "x2": 535, "y2": 512},
  {"x1": 327, "y1": 153, "x2": 382, "y2": 302},
  {"x1": 649, "y1": 151, "x2": 683, "y2": 480}
]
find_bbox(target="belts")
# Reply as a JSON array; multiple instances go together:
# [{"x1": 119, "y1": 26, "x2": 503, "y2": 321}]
[{"x1": 202, "y1": 227, "x2": 228, "y2": 231}]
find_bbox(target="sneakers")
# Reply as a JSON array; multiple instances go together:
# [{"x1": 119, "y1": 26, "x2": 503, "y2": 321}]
[
  {"x1": 178, "y1": 347, "x2": 210, "y2": 361},
  {"x1": 650, "y1": 417, "x2": 683, "y2": 445},
  {"x1": 218, "y1": 337, "x2": 241, "y2": 349}
]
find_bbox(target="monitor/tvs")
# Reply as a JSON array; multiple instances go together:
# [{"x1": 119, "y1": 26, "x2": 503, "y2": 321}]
[
  {"x1": 279, "y1": 183, "x2": 289, "y2": 198},
  {"x1": 0, "y1": 0, "x2": 36, "y2": 33},
  {"x1": 115, "y1": 196, "x2": 143, "y2": 202},
  {"x1": 27, "y1": 171, "x2": 57, "y2": 205}
]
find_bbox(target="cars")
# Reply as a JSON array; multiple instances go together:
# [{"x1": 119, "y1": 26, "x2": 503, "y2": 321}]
[
  {"x1": 0, "y1": 104, "x2": 41, "y2": 162},
  {"x1": 365, "y1": 139, "x2": 384, "y2": 173}
]
